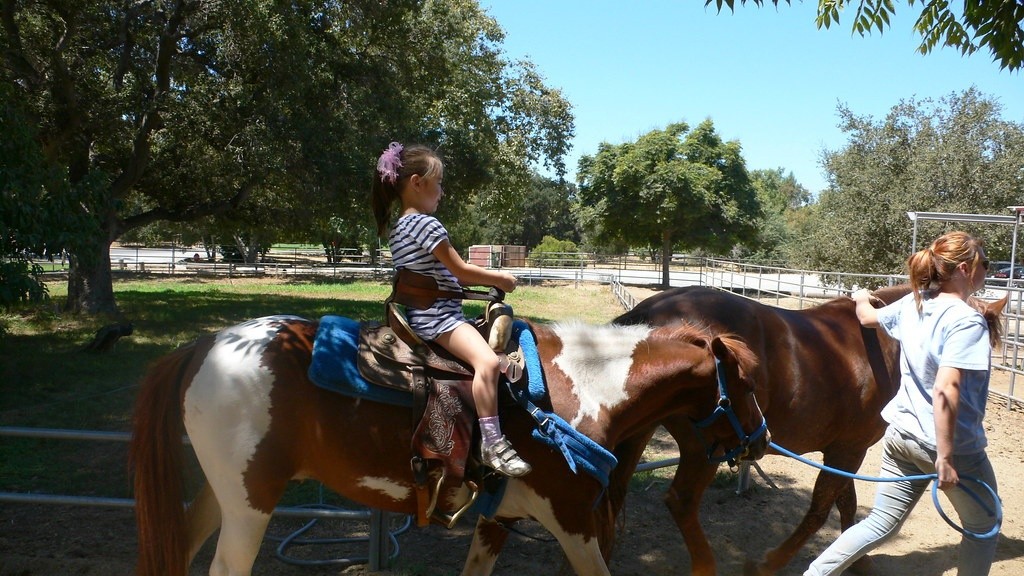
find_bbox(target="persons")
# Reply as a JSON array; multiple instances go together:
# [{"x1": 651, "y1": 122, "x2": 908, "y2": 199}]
[
  {"x1": 803, "y1": 231, "x2": 1003, "y2": 576},
  {"x1": 378, "y1": 142, "x2": 531, "y2": 479}
]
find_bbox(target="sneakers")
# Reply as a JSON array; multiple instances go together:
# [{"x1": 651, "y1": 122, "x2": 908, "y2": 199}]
[{"x1": 480, "y1": 434, "x2": 533, "y2": 478}]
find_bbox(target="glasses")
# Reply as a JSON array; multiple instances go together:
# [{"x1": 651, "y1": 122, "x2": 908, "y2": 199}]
[{"x1": 955, "y1": 259, "x2": 989, "y2": 270}]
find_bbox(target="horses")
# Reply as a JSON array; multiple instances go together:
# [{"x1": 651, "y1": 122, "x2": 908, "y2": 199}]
[
  {"x1": 595, "y1": 286, "x2": 1008, "y2": 576},
  {"x1": 127, "y1": 315, "x2": 771, "y2": 576}
]
